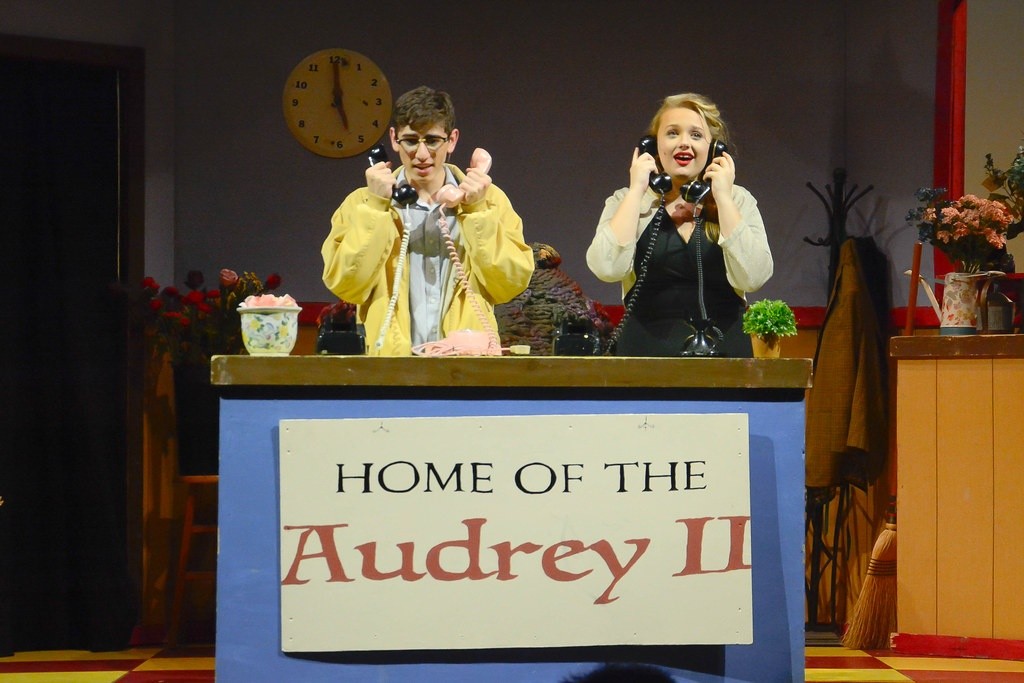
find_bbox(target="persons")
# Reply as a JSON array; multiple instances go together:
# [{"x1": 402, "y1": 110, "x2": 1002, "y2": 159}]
[
  {"x1": 585, "y1": 93, "x2": 775, "y2": 357},
  {"x1": 319, "y1": 86, "x2": 534, "y2": 357}
]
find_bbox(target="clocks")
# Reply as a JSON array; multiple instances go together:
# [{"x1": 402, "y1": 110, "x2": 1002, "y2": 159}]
[{"x1": 282, "y1": 48, "x2": 393, "y2": 158}]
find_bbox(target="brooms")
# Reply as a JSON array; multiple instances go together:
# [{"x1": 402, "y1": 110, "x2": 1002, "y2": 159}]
[{"x1": 842, "y1": 241, "x2": 923, "y2": 650}]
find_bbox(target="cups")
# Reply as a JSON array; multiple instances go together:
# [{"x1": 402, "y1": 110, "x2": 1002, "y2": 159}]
[{"x1": 238, "y1": 307, "x2": 302, "y2": 356}]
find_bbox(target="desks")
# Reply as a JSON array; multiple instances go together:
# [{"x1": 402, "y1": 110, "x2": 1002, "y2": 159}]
[{"x1": 211, "y1": 354, "x2": 814, "y2": 683}]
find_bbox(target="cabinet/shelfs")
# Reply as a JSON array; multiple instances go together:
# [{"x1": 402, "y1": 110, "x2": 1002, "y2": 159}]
[{"x1": 888, "y1": 335, "x2": 1024, "y2": 662}]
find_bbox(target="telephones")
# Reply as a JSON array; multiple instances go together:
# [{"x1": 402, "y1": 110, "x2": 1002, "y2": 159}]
[
  {"x1": 638, "y1": 133, "x2": 673, "y2": 196},
  {"x1": 435, "y1": 148, "x2": 492, "y2": 207},
  {"x1": 680, "y1": 140, "x2": 728, "y2": 202},
  {"x1": 367, "y1": 144, "x2": 417, "y2": 209}
]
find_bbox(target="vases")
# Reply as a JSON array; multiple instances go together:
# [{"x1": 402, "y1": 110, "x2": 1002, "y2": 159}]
[
  {"x1": 235, "y1": 306, "x2": 302, "y2": 357},
  {"x1": 316, "y1": 325, "x2": 364, "y2": 355},
  {"x1": 940, "y1": 270, "x2": 1008, "y2": 335},
  {"x1": 167, "y1": 361, "x2": 218, "y2": 476}
]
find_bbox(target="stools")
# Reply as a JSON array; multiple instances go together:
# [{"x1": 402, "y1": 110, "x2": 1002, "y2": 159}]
[{"x1": 166, "y1": 476, "x2": 219, "y2": 653}]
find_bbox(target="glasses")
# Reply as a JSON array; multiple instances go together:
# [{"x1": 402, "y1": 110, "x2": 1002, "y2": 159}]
[{"x1": 395, "y1": 133, "x2": 449, "y2": 152}]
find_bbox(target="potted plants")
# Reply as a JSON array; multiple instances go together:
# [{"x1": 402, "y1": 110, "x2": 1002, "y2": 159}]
[{"x1": 744, "y1": 299, "x2": 798, "y2": 358}]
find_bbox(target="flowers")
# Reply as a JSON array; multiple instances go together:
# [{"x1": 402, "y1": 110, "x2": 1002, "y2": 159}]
[
  {"x1": 903, "y1": 128, "x2": 1024, "y2": 274},
  {"x1": 315, "y1": 298, "x2": 356, "y2": 328},
  {"x1": 104, "y1": 269, "x2": 281, "y2": 364},
  {"x1": 239, "y1": 293, "x2": 299, "y2": 306}
]
